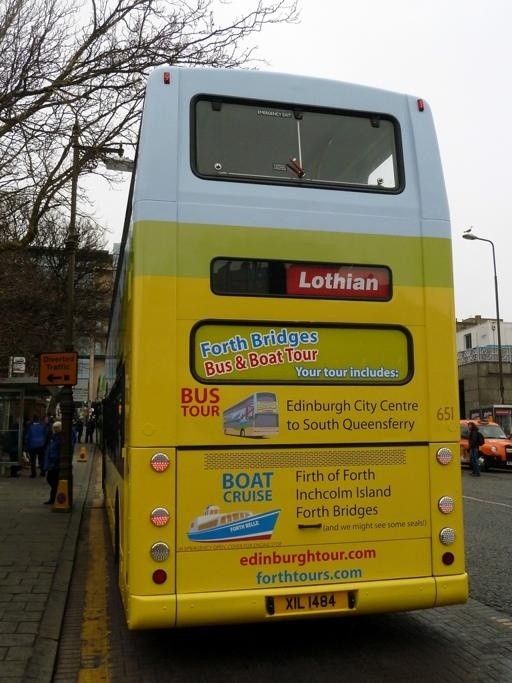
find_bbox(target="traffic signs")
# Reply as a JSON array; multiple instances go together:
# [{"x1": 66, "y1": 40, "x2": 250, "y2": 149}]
[
  {"x1": 52, "y1": 117, "x2": 137, "y2": 506},
  {"x1": 40, "y1": 351, "x2": 78, "y2": 385}
]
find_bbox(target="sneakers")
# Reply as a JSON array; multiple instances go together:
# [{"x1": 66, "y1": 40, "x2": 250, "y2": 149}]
[
  {"x1": 8, "y1": 465, "x2": 47, "y2": 479},
  {"x1": 469, "y1": 472, "x2": 481, "y2": 477},
  {"x1": 44, "y1": 500, "x2": 55, "y2": 504}
]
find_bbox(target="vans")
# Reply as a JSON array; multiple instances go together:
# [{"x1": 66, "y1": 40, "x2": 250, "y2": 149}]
[{"x1": 457, "y1": 414, "x2": 511, "y2": 467}]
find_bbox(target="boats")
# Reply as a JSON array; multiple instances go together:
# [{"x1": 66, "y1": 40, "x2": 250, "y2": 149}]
[{"x1": 182, "y1": 503, "x2": 281, "y2": 543}]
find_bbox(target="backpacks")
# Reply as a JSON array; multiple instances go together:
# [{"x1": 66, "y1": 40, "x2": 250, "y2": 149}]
[{"x1": 477, "y1": 432, "x2": 484, "y2": 446}]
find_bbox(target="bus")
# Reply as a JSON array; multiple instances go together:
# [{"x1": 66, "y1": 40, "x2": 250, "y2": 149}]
[
  {"x1": 467, "y1": 404, "x2": 511, "y2": 437},
  {"x1": 98, "y1": 64, "x2": 470, "y2": 628},
  {"x1": 219, "y1": 390, "x2": 278, "y2": 438}
]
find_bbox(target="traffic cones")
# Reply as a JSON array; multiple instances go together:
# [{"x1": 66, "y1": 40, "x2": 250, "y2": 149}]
[
  {"x1": 77, "y1": 446, "x2": 88, "y2": 461},
  {"x1": 51, "y1": 477, "x2": 72, "y2": 513}
]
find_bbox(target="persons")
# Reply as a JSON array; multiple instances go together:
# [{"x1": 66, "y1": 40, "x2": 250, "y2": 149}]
[
  {"x1": 0, "y1": 404, "x2": 96, "y2": 505},
  {"x1": 467, "y1": 422, "x2": 480, "y2": 476}
]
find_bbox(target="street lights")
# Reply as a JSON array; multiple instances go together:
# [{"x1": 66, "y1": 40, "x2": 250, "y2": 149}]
[{"x1": 462, "y1": 230, "x2": 506, "y2": 404}]
